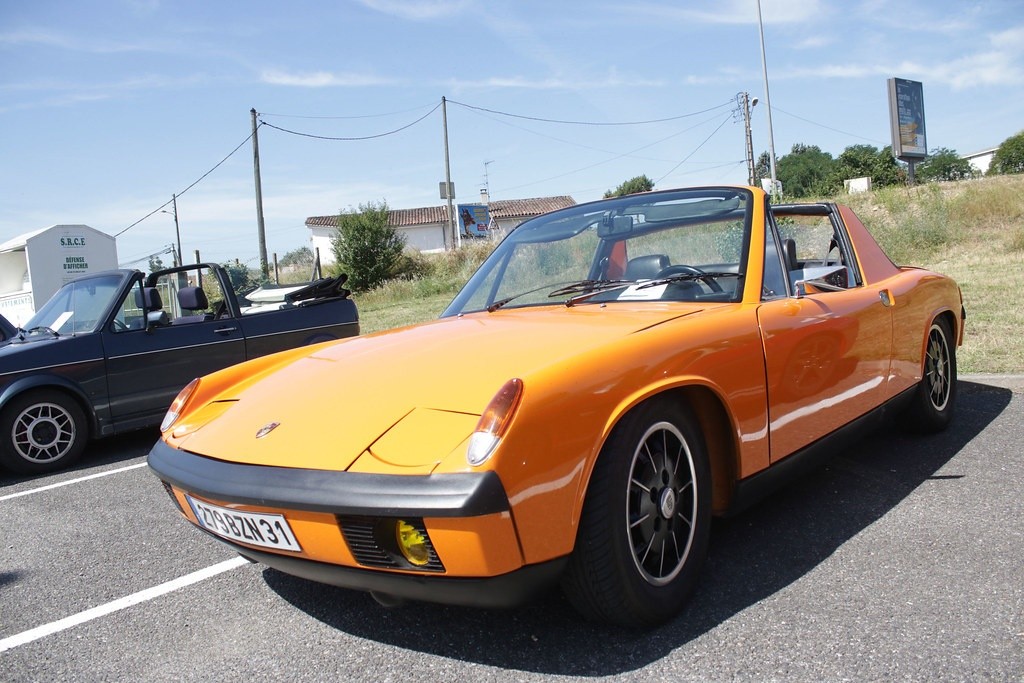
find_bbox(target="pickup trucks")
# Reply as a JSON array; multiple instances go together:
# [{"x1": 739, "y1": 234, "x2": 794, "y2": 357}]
[{"x1": 0, "y1": 260, "x2": 362, "y2": 480}]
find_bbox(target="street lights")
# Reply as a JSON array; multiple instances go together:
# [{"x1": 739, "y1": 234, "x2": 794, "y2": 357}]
[
  {"x1": 738, "y1": 97, "x2": 758, "y2": 185},
  {"x1": 161, "y1": 209, "x2": 182, "y2": 266}
]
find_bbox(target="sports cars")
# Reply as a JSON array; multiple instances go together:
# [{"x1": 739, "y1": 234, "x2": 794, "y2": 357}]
[{"x1": 143, "y1": 183, "x2": 968, "y2": 633}]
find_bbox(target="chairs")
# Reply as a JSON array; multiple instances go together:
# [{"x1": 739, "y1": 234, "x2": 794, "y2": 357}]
[
  {"x1": 764, "y1": 239, "x2": 798, "y2": 295},
  {"x1": 172, "y1": 286, "x2": 214, "y2": 325},
  {"x1": 624, "y1": 254, "x2": 670, "y2": 281},
  {"x1": 129, "y1": 287, "x2": 168, "y2": 330}
]
